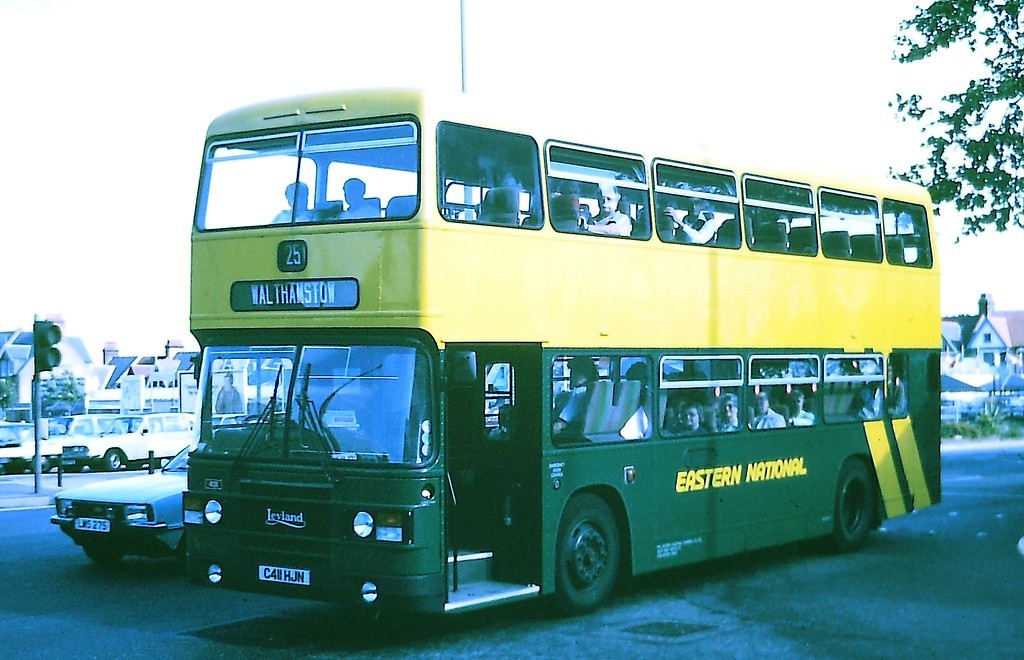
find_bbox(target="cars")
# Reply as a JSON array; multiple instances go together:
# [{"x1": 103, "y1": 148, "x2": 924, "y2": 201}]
[{"x1": 50, "y1": 443, "x2": 195, "y2": 561}]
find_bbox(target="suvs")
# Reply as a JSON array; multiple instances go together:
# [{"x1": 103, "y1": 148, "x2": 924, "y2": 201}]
[{"x1": 62, "y1": 413, "x2": 195, "y2": 472}]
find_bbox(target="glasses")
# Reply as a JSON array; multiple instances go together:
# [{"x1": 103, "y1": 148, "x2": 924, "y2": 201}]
[{"x1": 724, "y1": 403, "x2": 735, "y2": 411}]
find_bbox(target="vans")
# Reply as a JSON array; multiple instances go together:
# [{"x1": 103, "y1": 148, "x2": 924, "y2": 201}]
[{"x1": 0, "y1": 414, "x2": 120, "y2": 476}]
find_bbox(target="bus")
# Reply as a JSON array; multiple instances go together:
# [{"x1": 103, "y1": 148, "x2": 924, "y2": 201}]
[{"x1": 181, "y1": 87, "x2": 941, "y2": 616}]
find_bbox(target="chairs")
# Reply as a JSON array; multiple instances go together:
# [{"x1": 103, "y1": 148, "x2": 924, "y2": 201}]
[
  {"x1": 578, "y1": 378, "x2": 859, "y2": 442},
  {"x1": 312, "y1": 186, "x2": 906, "y2": 269}
]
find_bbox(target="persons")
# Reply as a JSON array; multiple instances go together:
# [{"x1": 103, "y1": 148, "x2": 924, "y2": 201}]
[
  {"x1": 558, "y1": 179, "x2": 717, "y2": 244},
  {"x1": 488, "y1": 404, "x2": 513, "y2": 439},
  {"x1": 215, "y1": 374, "x2": 243, "y2": 415},
  {"x1": 270, "y1": 181, "x2": 312, "y2": 223},
  {"x1": 552, "y1": 356, "x2": 905, "y2": 439},
  {"x1": 335, "y1": 178, "x2": 380, "y2": 219}
]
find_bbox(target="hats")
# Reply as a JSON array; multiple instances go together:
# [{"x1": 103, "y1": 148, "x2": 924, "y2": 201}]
[{"x1": 720, "y1": 392, "x2": 739, "y2": 407}]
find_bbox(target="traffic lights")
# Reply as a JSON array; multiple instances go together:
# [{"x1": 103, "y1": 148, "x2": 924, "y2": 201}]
[{"x1": 34, "y1": 321, "x2": 61, "y2": 372}]
